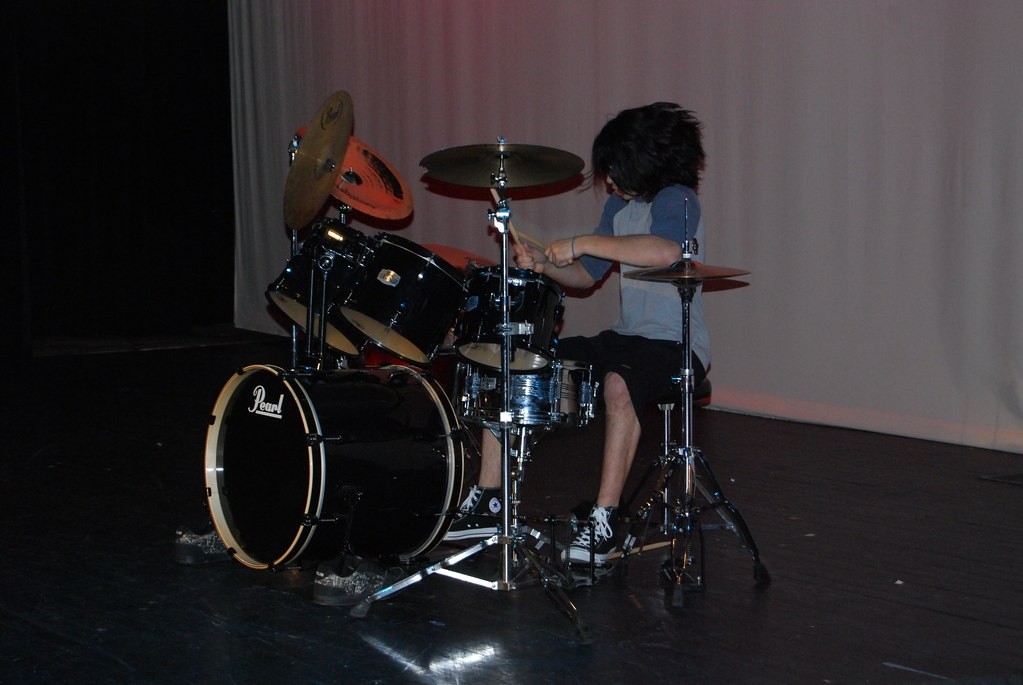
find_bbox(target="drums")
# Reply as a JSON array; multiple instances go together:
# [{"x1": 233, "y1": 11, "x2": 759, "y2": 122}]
[
  {"x1": 203, "y1": 364, "x2": 466, "y2": 572},
  {"x1": 340, "y1": 231, "x2": 470, "y2": 363},
  {"x1": 451, "y1": 357, "x2": 598, "y2": 428},
  {"x1": 453, "y1": 264, "x2": 565, "y2": 371},
  {"x1": 267, "y1": 217, "x2": 378, "y2": 355}
]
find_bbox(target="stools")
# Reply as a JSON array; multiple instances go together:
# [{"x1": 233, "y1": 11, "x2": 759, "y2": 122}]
[{"x1": 622, "y1": 376, "x2": 743, "y2": 541}]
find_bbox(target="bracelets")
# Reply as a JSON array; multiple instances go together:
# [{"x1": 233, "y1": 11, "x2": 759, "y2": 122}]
[{"x1": 572, "y1": 238, "x2": 575, "y2": 256}]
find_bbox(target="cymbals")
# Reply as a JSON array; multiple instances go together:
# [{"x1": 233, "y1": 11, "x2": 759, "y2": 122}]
[
  {"x1": 419, "y1": 243, "x2": 496, "y2": 267},
  {"x1": 295, "y1": 128, "x2": 412, "y2": 220},
  {"x1": 283, "y1": 90, "x2": 355, "y2": 230},
  {"x1": 419, "y1": 143, "x2": 586, "y2": 188},
  {"x1": 623, "y1": 259, "x2": 749, "y2": 290}
]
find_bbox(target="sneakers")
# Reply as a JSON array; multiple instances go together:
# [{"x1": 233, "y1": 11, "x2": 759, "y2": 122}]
[
  {"x1": 441, "y1": 486, "x2": 504, "y2": 541},
  {"x1": 560, "y1": 502, "x2": 619, "y2": 565}
]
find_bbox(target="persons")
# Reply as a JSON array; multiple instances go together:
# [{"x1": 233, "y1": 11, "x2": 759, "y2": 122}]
[{"x1": 441, "y1": 101, "x2": 707, "y2": 564}]
